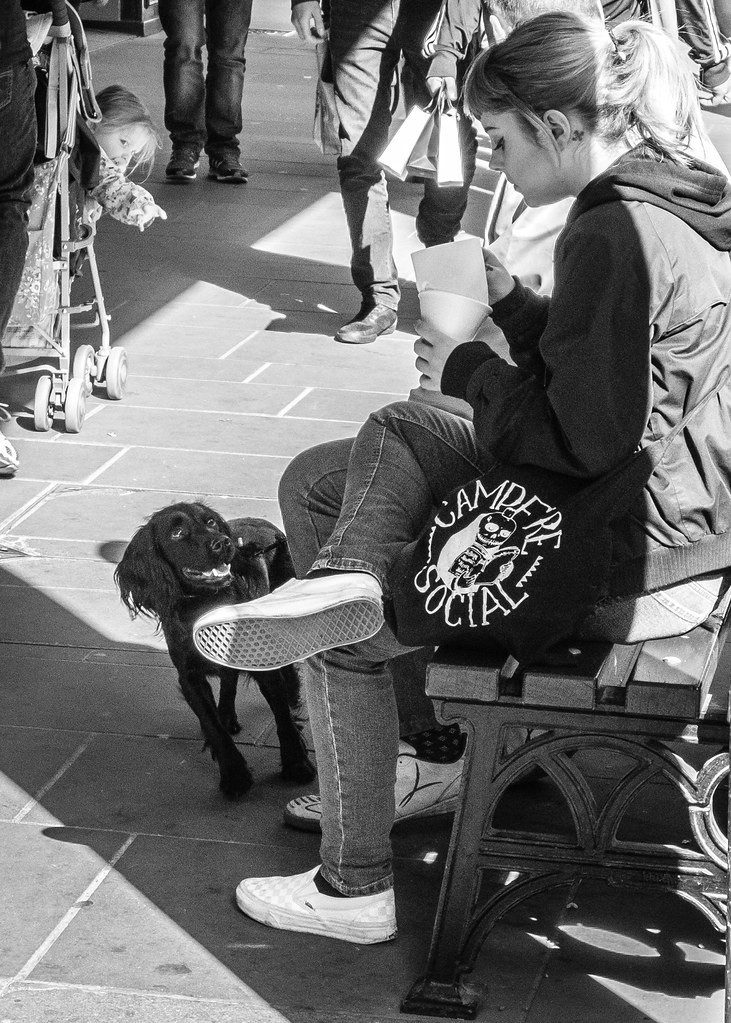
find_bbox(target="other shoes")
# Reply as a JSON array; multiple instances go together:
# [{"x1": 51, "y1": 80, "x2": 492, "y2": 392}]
[{"x1": 0, "y1": 404, "x2": 20, "y2": 475}]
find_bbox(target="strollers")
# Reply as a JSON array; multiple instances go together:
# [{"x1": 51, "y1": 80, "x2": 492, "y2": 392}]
[{"x1": 0, "y1": 0, "x2": 128, "y2": 435}]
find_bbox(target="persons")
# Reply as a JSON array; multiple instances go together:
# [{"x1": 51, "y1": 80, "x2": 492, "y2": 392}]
[{"x1": 0, "y1": 0, "x2": 731, "y2": 943}]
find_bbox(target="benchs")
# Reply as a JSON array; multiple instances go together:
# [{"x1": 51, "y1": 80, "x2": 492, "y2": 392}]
[{"x1": 400, "y1": 586, "x2": 731, "y2": 1018}]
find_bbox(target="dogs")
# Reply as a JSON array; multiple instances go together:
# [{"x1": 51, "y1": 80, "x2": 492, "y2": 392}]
[{"x1": 113, "y1": 502, "x2": 317, "y2": 798}]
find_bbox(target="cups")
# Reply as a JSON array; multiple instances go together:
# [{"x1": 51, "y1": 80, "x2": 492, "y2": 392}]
[{"x1": 417, "y1": 289, "x2": 493, "y2": 346}]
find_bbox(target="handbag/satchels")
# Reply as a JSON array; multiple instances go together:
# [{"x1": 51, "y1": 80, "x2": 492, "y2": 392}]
[{"x1": 375, "y1": 87, "x2": 471, "y2": 188}]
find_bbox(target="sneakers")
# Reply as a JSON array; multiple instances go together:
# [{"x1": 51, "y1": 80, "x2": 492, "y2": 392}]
[
  {"x1": 236, "y1": 865, "x2": 397, "y2": 945},
  {"x1": 284, "y1": 732, "x2": 467, "y2": 832},
  {"x1": 193, "y1": 574, "x2": 386, "y2": 672},
  {"x1": 165, "y1": 145, "x2": 249, "y2": 184},
  {"x1": 335, "y1": 301, "x2": 399, "y2": 344}
]
